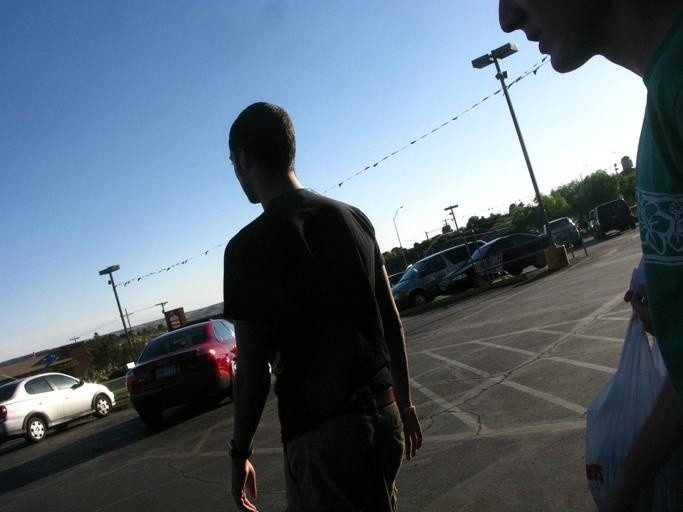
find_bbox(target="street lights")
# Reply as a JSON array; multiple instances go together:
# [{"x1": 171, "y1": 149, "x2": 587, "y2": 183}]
[
  {"x1": 99, "y1": 264, "x2": 137, "y2": 364},
  {"x1": 470, "y1": 41, "x2": 556, "y2": 248}
]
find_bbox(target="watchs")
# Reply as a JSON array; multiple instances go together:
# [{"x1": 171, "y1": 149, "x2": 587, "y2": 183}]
[{"x1": 226, "y1": 440, "x2": 254, "y2": 460}]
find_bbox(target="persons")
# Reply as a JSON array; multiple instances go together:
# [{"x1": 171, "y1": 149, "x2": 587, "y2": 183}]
[
  {"x1": 225, "y1": 102, "x2": 422, "y2": 512},
  {"x1": 499, "y1": 1, "x2": 681, "y2": 512}
]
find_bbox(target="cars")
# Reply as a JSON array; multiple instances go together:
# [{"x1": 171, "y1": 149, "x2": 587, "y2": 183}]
[
  {"x1": 384, "y1": 197, "x2": 641, "y2": 309},
  {"x1": 125, "y1": 316, "x2": 273, "y2": 428},
  {"x1": 0, "y1": 369, "x2": 118, "y2": 445}
]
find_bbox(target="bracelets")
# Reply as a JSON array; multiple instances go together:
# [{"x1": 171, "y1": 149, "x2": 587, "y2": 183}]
[{"x1": 398, "y1": 406, "x2": 417, "y2": 414}]
